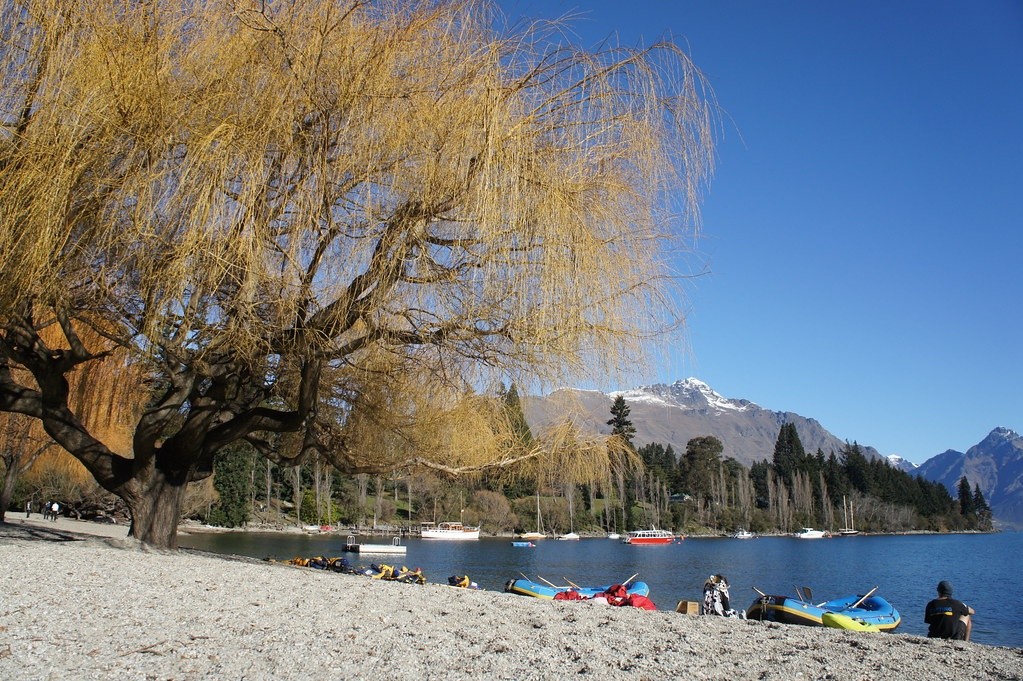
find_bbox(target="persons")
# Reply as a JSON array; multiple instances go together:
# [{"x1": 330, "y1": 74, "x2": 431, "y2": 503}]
[
  {"x1": 924, "y1": 580, "x2": 975, "y2": 642},
  {"x1": 26, "y1": 500, "x2": 33, "y2": 518},
  {"x1": 50, "y1": 501, "x2": 60, "y2": 522},
  {"x1": 42, "y1": 502, "x2": 48, "y2": 519}
]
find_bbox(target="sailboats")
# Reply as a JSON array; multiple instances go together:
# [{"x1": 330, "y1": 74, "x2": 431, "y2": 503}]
[{"x1": 838, "y1": 494, "x2": 859, "y2": 535}]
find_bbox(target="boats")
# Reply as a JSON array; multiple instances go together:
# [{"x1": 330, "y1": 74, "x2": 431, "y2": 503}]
[
  {"x1": 505, "y1": 571, "x2": 650, "y2": 601},
  {"x1": 726, "y1": 527, "x2": 759, "y2": 539},
  {"x1": 790, "y1": 527, "x2": 826, "y2": 538},
  {"x1": 420, "y1": 522, "x2": 482, "y2": 539},
  {"x1": 619, "y1": 523, "x2": 676, "y2": 545},
  {"x1": 747, "y1": 586, "x2": 902, "y2": 633},
  {"x1": 555, "y1": 532, "x2": 580, "y2": 541},
  {"x1": 342, "y1": 536, "x2": 407, "y2": 554},
  {"x1": 520, "y1": 533, "x2": 548, "y2": 539},
  {"x1": 608, "y1": 533, "x2": 622, "y2": 540},
  {"x1": 511, "y1": 542, "x2": 536, "y2": 548}
]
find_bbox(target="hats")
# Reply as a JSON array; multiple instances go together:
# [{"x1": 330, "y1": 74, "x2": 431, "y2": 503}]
[{"x1": 937, "y1": 581, "x2": 953, "y2": 596}]
